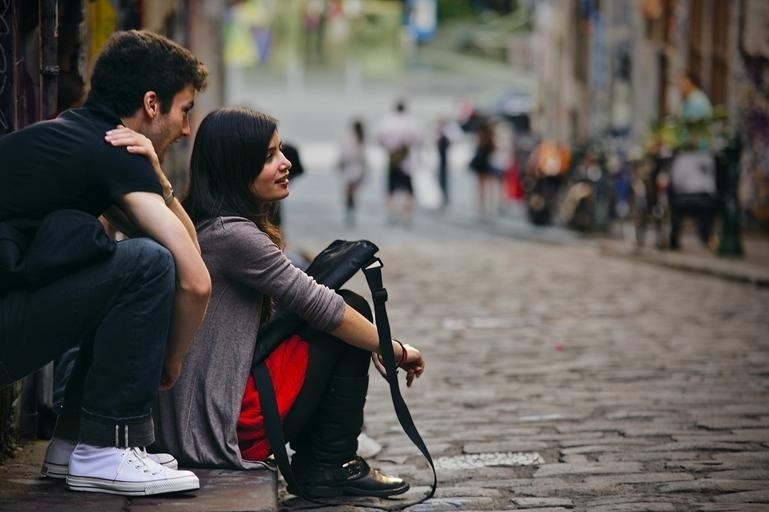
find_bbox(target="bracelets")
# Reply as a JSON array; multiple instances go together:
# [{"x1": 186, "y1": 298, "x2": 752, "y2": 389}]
[
  {"x1": 377, "y1": 339, "x2": 405, "y2": 370},
  {"x1": 164, "y1": 189, "x2": 175, "y2": 206}
]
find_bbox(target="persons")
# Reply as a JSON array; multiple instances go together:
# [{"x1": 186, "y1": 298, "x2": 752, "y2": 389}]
[
  {"x1": 340, "y1": 100, "x2": 534, "y2": 222},
  {"x1": 661, "y1": 67, "x2": 719, "y2": 251},
  {"x1": 147, "y1": 108, "x2": 425, "y2": 496},
  {"x1": 0, "y1": 30, "x2": 212, "y2": 496}
]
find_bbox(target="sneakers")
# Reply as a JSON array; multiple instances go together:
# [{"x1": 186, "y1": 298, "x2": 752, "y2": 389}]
[{"x1": 41, "y1": 435, "x2": 200, "y2": 496}]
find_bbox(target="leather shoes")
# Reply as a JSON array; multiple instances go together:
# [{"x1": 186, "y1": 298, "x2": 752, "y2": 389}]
[{"x1": 287, "y1": 454, "x2": 410, "y2": 498}]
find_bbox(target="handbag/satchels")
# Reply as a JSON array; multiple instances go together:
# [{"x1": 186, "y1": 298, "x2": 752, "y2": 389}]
[{"x1": 252, "y1": 240, "x2": 384, "y2": 369}]
